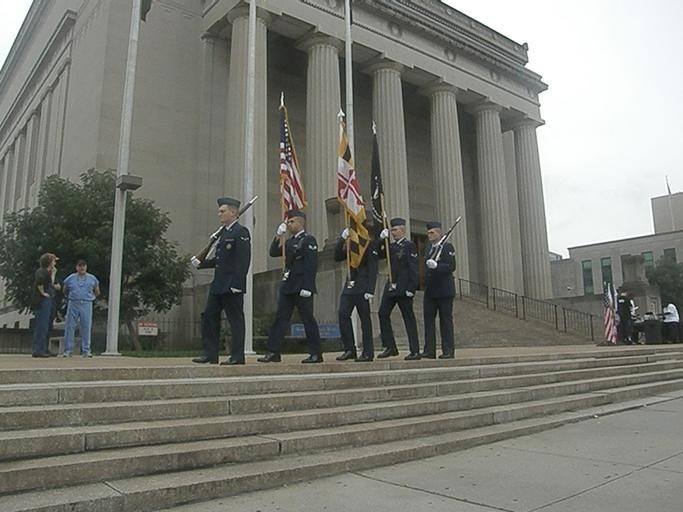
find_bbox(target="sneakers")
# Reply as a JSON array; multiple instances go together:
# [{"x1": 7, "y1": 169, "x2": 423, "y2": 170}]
[
  {"x1": 63, "y1": 350, "x2": 72, "y2": 357},
  {"x1": 82, "y1": 352, "x2": 92, "y2": 358}
]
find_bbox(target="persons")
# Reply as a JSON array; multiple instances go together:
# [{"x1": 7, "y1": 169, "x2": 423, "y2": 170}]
[
  {"x1": 374, "y1": 218, "x2": 420, "y2": 360},
  {"x1": 256, "y1": 209, "x2": 323, "y2": 363},
  {"x1": 58, "y1": 259, "x2": 100, "y2": 359},
  {"x1": 190, "y1": 197, "x2": 251, "y2": 365},
  {"x1": 334, "y1": 217, "x2": 378, "y2": 362},
  {"x1": 654, "y1": 297, "x2": 679, "y2": 344},
  {"x1": 31, "y1": 251, "x2": 60, "y2": 359},
  {"x1": 626, "y1": 292, "x2": 641, "y2": 345},
  {"x1": 418, "y1": 222, "x2": 458, "y2": 358},
  {"x1": 616, "y1": 286, "x2": 632, "y2": 345}
]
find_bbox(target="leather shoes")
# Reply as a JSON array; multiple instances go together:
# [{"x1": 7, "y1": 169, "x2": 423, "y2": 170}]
[
  {"x1": 32, "y1": 351, "x2": 57, "y2": 357},
  {"x1": 257, "y1": 352, "x2": 281, "y2": 362},
  {"x1": 377, "y1": 344, "x2": 399, "y2": 358},
  {"x1": 405, "y1": 353, "x2": 421, "y2": 360},
  {"x1": 355, "y1": 351, "x2": 373, "y2": 362},
  {"x1": 220, "y1": 357, "x2": 245, "y2": 365},
  {"x1": 192, "y1": 356, "x2": 218, "y2": 364},
  {"x1": 336, "y1": 349, "x2": 357, "y2": 361},
  {"x1": 421, "y1": 352, "x2": 436, "y2": 358},
  {"x1": 302, "y1": 353, "x2": 323, "y2": 363},
  {"x1": 439, "y1": 354, "x2": 455, "y2": 359}
]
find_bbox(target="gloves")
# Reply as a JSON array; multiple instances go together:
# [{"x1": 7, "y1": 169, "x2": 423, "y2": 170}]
[
  {"x1": 406, "y1": 291, "x2": 414, "y2": 297},
  {"x1": 231, "y1": 287, "x2": 243, "y2": 294},
  {"x1": 277, "y1": 222, "x2": 287, "y2": 236},
  {"x1": 300, "y1": 289, "x2": 311, "y2": 298},
  {"x1": 341, "y1": 227, "x2": 349, "y2": 240},
  {"x1": 364, "y1": 293, "x2": 374, "y2": 300},
  {"x1": 191, "y1": 256, "x2": 201, "y2": 269},
  {"x1": 426, "y1": 259, "x2": 438, "y2": 269},
  {"x1": 380, "y1": 229, "x2": 389, "y2": 239}
]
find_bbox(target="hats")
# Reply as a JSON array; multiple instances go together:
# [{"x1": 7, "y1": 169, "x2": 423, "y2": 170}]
[
  {"x1": 426, "y1": 222, "x2": 441, "y2": 229},
  {"x1": 287, "y1": 209, "x2": 307, "y2": 219},
  {"x1": 390, "y1": 218, "x2": 405, "y2": 227},
  {"x1": 217, "y1": 197, "x2": 241, "y2": 208}
]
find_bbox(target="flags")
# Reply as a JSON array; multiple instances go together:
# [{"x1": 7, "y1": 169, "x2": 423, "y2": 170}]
[
  {"x1": 602, "y1": 281, "x2": 617, "y2": 344},
  {"x1": 335, "y1": 107, "x2": 371, "y2": 270},
  {"x1": 369, "y1": 119, "x2": 391, "y2": 260},
  {"x1": 278, "y1": 88, "x2": 307, "y2": 228}
]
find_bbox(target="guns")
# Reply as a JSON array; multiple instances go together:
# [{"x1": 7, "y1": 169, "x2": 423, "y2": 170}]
[
  {"x1": 195, "y1": 195, "x2": 259, "y2": 264},
  {"x1": 430, "y1": 216, "x2": 462, "y2": 263}
]
